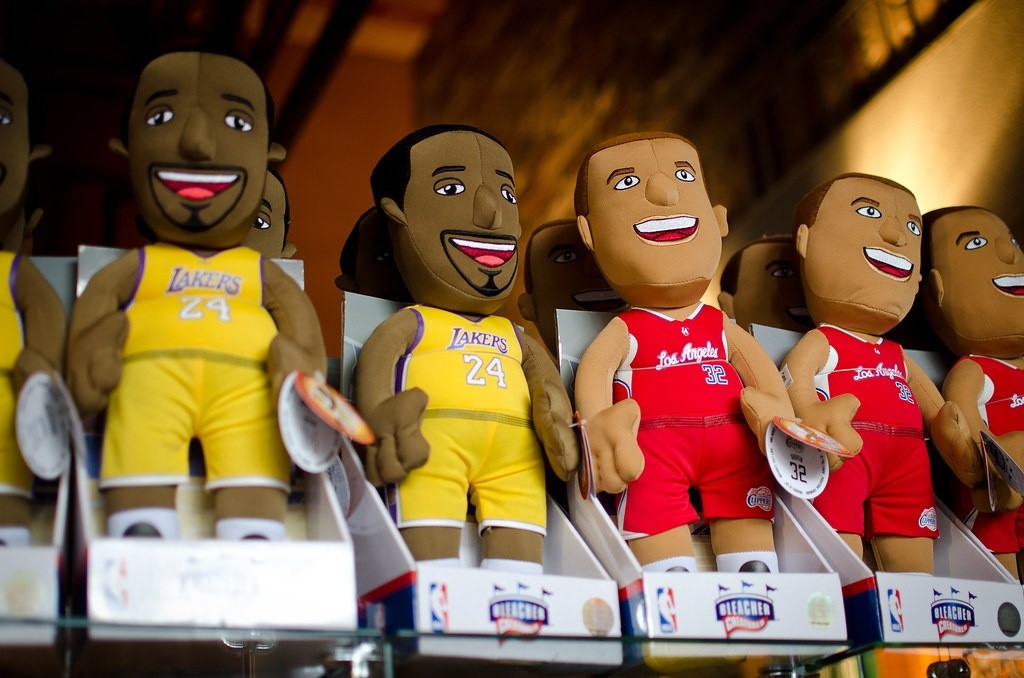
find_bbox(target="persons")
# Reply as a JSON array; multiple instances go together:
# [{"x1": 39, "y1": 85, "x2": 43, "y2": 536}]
[
  {"x1": 518, "y1": 219, "x2": 633, "y2": 365},
  {"x1": 0, "y1": 53, "x2": 66, "y2": 554},
  {"x1": 717, "y1": 232, "x2": 815, "y2": 335},
  {"x1": 355, "y1": 123, "x2": 578, "y2": 572},
  {"x1": 242, "y1": 165, "x2": 298, "y2": 260},
  {"x1": 336, "y1": 205, "x2": 416, "y2": 304},
  {"x1": 918, "y1": 205, "x2": 1023, "y2": 584},
  {"x1": 575, "y1": 131, "x2": 797, "y2": 572},
  {"x1": 3, "y1": 185, "x2": 44, "y2": 257},
  {"x1": 67, "y1": 40, "x2": 320, "y2": 540},
  {"x1": 781, "y1": 172, "x2": 984, "y2": 574}
]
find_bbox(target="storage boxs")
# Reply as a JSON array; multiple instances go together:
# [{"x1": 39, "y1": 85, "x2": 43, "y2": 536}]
[{"x1": 0, "y1": 244, "x2": 1024, "y2": 648}]
